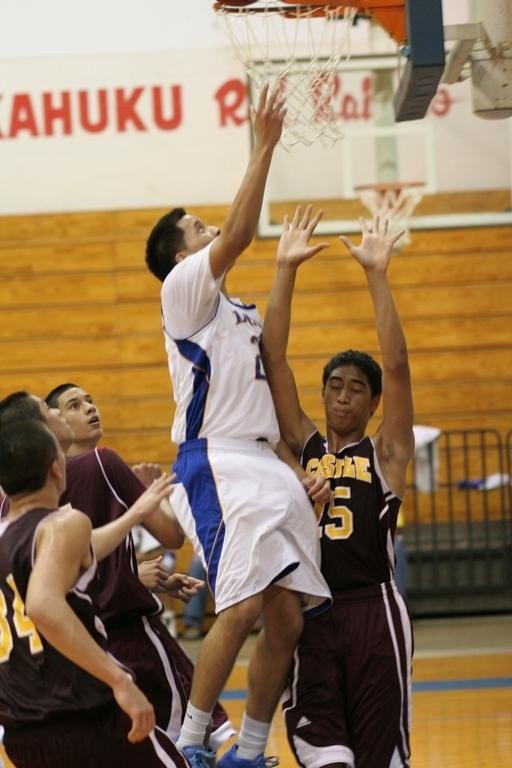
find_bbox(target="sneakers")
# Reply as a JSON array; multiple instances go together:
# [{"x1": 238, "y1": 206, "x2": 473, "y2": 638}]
[
  {"x1": 215, "y1": 743, "x2": 279, "y2": 768},
  {"x1": 179, "y1": 745, "x2": 215, "y2": 768}
]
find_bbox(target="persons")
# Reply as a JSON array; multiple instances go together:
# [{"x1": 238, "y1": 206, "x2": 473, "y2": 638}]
[
  {"x1": 260, "y1": 204, "x2": 414, "y2": 768},
  {"x1": 145, "y1": 80, "x2": 334, "y2": 768},
  {"x1": 0, "y1": 383, "x2": 233, "y2": 768}
]
